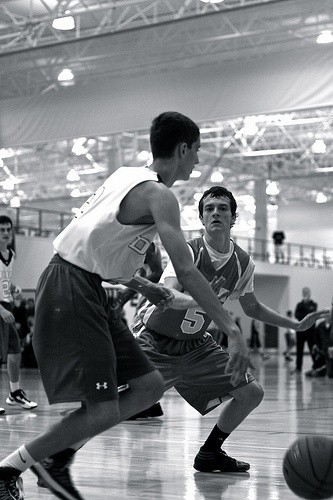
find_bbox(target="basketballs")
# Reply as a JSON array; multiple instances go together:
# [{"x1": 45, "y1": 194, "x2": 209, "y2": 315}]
[{"x1": 282, "y1": 433, "x2": 333, "y2": 500}]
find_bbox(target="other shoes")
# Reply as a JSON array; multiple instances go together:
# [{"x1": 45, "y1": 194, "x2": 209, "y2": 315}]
[{"x1": 305, "y1": 370, "x2": 326, "y2": 378}]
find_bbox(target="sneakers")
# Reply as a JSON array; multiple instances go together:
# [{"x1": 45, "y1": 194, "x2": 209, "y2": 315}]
[
  {"x1": 6, "y1": 389, "x2": 38, "y2": 410},
  {"x1": 29, "y1": 448, "x2": 86, "y2": 500},
  {"x1": 0, "y1": 408, "x2": 5, "y2": 414},
  {"x1": 193, "y1": 446, "x2": 250, "y2": 472},
  {"x1": 0, "y1": 466, "x2": 25, "y2": 500}
]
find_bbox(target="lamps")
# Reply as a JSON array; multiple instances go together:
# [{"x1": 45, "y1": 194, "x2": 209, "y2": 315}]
[
  {"x1": 316, "y1": 29, "x2": 333, "y2": 44},
  {"x1": 58, "y1": 65, "x2": 75, "y2": 87},
  {"x1": 52, "y1": 5, "x2": 76, "y2": 31}
]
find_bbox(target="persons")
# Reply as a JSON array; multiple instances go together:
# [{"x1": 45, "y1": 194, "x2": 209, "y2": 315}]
[
  {"x1": 284, "y1": 311, "x2": 296, "y2": 361},
  {"x1": 129, "y1": 186, "x2": 333, "y2": 472},
  {"x1": 0, "y1": 112, "x2": 256, "y2": 500},
  {"x1": 248, "y1": 319, "x2": 261, "y2": 353},
  {"x1": 0, "y1": 215, "x2": 38, "y2": 414},
  {"x1": 11, "y1": 298, "x2": 39, "y2": 370},
  {"x1": 101, "y1": 239, "x2": 165, "y2": 421},
  {"x1": 304, "y1": 308, "x2": 333, "y2": 378},
  {"x1": 292, "y1": 287, "x2": 318, "y2": 373},
  {"x1": 213, "y1": 308, "x2": 241, "y2": 349}
]
